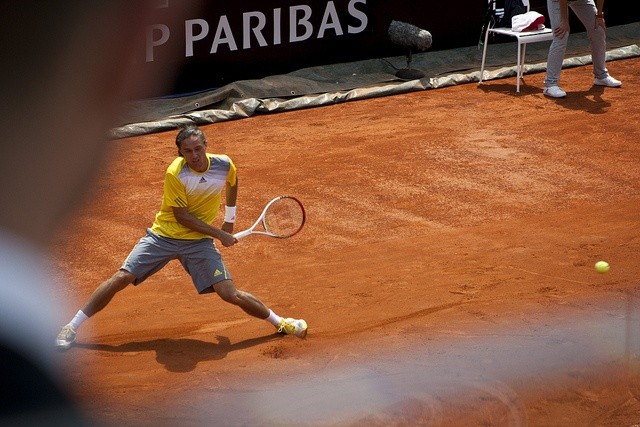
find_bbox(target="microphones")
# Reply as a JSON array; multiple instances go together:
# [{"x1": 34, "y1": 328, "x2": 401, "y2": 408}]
[{"x1": 387, "y1": 20, "x2": 433, "y2": 51}]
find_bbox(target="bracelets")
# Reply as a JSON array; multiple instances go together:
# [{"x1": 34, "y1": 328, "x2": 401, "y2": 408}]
[
  {"x1": 223, "y1": 205, "x2": 237, "y2": 224},
  {"x1": 596, "y1": 12, "x2": 605, "y2": 18}
]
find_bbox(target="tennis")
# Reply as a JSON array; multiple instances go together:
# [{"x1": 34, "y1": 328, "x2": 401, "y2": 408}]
[{"x1": 595, "y1": 261, "x2": 610, "y2": 274}]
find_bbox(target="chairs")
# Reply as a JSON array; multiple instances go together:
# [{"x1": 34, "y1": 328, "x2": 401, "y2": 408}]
[{"x1": 479, "y1": 1, "x2": 553, "y2": 92}]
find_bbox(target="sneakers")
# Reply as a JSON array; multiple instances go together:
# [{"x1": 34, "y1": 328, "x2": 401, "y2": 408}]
[
  {"x1": 55, "y1": 324, "x2": 76, "y2": 347},
  {"x1": 544, "y1": 87, "x2": 566, "y2": 98},
  {"x1": 593, "y1": 77, "x2": 619, "y2": 88},
  {"x1": 277, "y1": 318, "x2": 308, "y2": 338}
]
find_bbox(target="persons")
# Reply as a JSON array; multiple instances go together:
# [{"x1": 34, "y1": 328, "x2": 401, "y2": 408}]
[
  {"x1": 55, "y1": 125, "x2": 308, "y2": 352},
  {"x1": 544, "y1": 0, "x2": 622, "y2": 97},
  {"x1": 0, "y1": 0, "x2": 184, "y2": 427}
]
